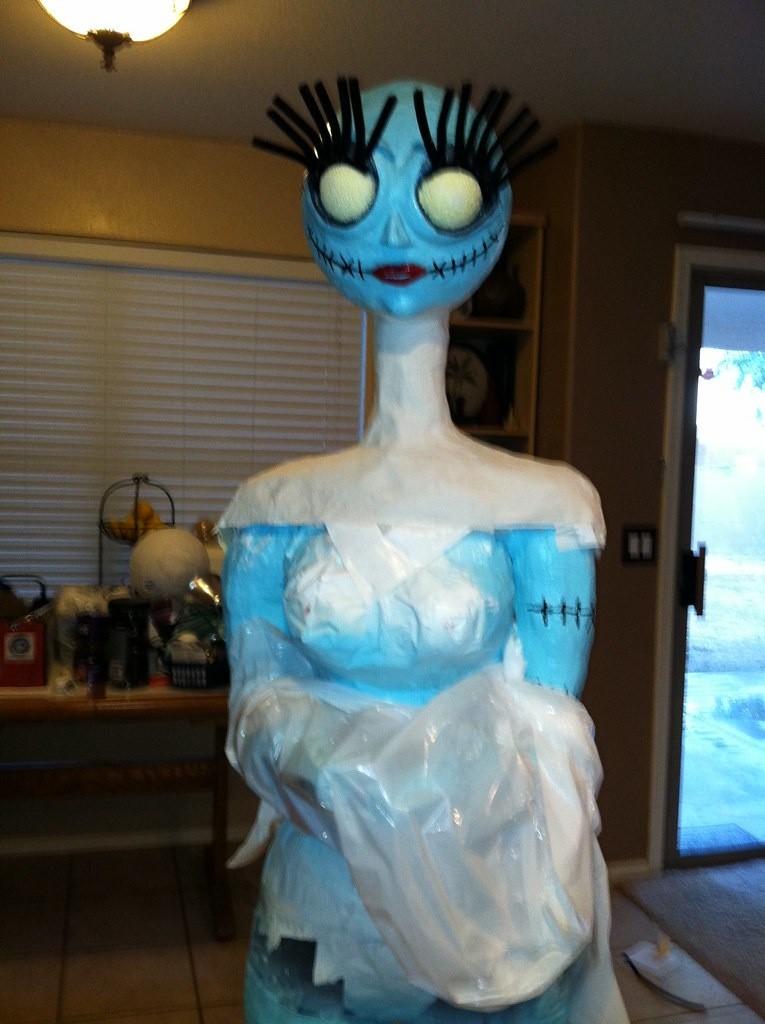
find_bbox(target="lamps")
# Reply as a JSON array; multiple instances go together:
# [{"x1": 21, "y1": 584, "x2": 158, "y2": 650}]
[{"x1": 35, "y1": 0, "x2": 192, "y2": 72}]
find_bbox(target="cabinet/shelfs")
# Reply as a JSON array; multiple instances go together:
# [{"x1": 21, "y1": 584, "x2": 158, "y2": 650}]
[{"x1": 448, "y1": 211, "x2": 548, "y2": 456}]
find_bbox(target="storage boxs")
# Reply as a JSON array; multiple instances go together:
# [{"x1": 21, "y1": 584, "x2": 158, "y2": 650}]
[{"x1": 0, "y1": 622, "x2": 47, "y2": 686}]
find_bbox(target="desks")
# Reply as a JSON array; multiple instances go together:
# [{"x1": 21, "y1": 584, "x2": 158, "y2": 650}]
[{"x1": 0, "y1": 687, "x2": 238, "y2": 941}]
